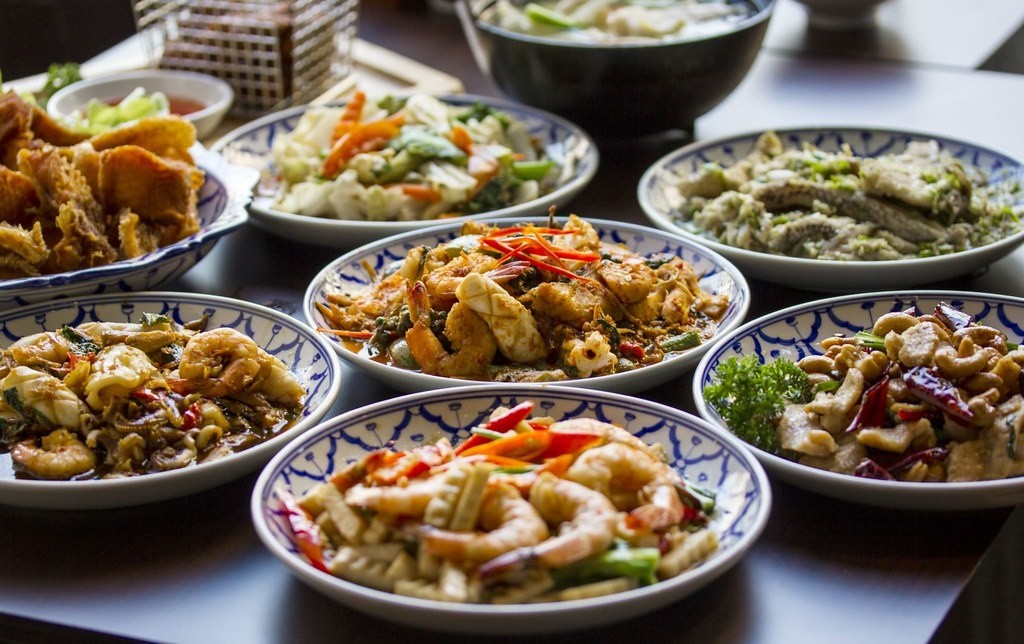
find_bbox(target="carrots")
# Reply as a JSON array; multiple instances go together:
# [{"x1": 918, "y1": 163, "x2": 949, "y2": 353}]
[{"x1": 320, "y1": 93, "x2": 473, "y2": 202}]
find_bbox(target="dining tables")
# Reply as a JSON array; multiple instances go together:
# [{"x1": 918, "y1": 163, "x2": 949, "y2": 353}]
[{"x1": 0, "y1": 0, "x2": 1024, "y2": 644}]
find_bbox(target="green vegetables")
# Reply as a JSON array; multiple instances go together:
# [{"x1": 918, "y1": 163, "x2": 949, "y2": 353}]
[
  {"x1": 702, "y1": 354, "x2": 812, "y2": 453},
  {"x1": 377, "y1": 94, "x2": 550, "y2": 214},
  {"x1": 40, "y1": 62, "x2": 155, "y2": 135}
]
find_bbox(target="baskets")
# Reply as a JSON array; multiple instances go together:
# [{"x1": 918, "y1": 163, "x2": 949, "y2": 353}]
[{"x1": 132, "y1": 0, "x2": 358, "y2": 117}]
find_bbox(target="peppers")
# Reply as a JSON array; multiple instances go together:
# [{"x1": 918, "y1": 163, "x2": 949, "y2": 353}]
[
  {"x1": 843, "y1": 302, "x2": 976, "y2": 483},
  {"x1": 280, "y1": 398, "x2": 603, "y2": 578}
]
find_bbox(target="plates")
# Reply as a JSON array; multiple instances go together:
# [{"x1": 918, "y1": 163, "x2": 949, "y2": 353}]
[
  {"x1": 251, "y1": 382, "x2": 771, "y2": 630},
  {"x1": 210, "y1": 90, "x2": 599, "y2": 247},
  {"x1": 694, "y1": 290, "x2": 1024, "y2": 509},
  {"x1": 303, "y1": 214, "x2": 750, "y2": 390},
  {"x1": 0, "y1": 290, "x2": 342, "y2": 513},
  {"x1": 637, "y1": 126, "x2": 1024, "y2": 286}
]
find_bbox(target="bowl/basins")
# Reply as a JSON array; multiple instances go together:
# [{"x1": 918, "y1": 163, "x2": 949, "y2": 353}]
[
  {"x1": 475, "y1": 0, "x2": 776, "y2": 136},
  {"x1": 0, "y1": 138, "x2": 249, "y2": 298},
  {"x1": 49, "y1": 68, "x2": 229, "y2": 139}
]
